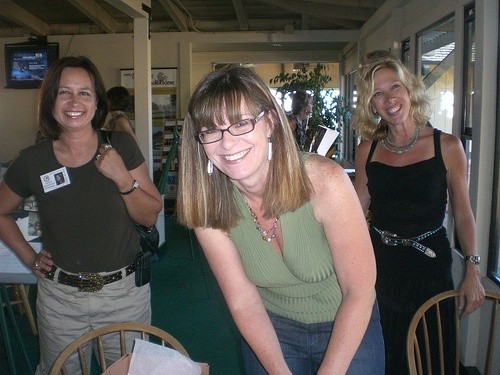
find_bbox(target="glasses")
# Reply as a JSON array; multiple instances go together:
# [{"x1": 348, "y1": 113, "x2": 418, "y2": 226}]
[{"x1": 194, "y1": 110, "x2": 266, "y2": 144}]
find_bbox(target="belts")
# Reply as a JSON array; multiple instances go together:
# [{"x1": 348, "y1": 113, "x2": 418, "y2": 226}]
[
  {"x1": 373, "y1": 226, "x2": 442, "y2": 258},
  {"x1": 40, "y1": 263, "x2": 137, "y2": 292}
]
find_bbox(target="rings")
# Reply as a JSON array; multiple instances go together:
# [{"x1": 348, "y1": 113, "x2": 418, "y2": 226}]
[
  {"x1": 35, "y1": 261, "x2": 40, "y2": 267},
  {"x1": 96, "y1": 154, "x2": 101, "y2": 160},
  {"x1": 104, "y1": 144, "x2": 112, "y2": 150}
]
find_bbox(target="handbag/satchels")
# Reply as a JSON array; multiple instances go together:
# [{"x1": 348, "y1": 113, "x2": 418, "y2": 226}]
[{"x1": 101, "y1": 129, "x2": 159, "y2": 256}]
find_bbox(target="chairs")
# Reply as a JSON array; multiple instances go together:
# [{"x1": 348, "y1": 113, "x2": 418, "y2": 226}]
[
  {"x1": 47, "y1": 321, "x2": 191, "y2": 375},
  {"x1": 406, "y1": 289, "x2": 500, "y2": 375}
]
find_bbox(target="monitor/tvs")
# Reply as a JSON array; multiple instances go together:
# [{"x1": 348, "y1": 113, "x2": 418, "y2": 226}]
[{"x1": 4, "y1": 42, "x2": 60, "y2": 89}]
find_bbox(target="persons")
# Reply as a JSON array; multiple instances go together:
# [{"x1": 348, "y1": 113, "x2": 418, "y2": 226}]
[
  {"x1": 353, "y1": 54, "x2": 485, "y2": 375},
  {"x1": 106, "y1": 86, "x2": 137, "y2": 141},
  {"x1": 288, "y1": 91, "x2": 312, "y2": 149},
  {"x1": 0, "y1": 56, "x2": 162, "y2": 375},
  {"x1": 24, "y1": 200, "x2": 42, "y2": 236},
  {"x1": 55, "y1": 174, "x2": 64, "y2": 185},
  {"x1": 176, "y1": 67, "x2": 386, "y2": 375}
]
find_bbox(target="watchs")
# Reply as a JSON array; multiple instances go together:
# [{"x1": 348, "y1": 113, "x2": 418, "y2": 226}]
[{"x1": 120, "y1": 180, "x2": 138, "y2": 195}]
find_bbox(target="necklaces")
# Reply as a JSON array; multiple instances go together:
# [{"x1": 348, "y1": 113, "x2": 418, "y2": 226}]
[
  {"x1": 381, "y1": 125, "x2": 420, "y2": 153},
  {"x1": 246, "y1": 201, "x2": 279, "y2": 241}
]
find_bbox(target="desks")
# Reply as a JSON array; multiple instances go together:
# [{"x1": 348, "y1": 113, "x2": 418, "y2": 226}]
[
  {"x1": 153, "y1": 194, "x2": 168, "y2": 261},
  {"x1": 0, "y1": 236, "x2": 43, "y2": 337},
  {"x1": 333, "y1": 157, "x2": 355, "y2": 174}
]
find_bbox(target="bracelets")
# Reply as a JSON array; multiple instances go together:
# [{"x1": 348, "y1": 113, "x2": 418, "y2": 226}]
[{"x1": 465, "y1": 255, "x2": 480, "y2": 264}]
